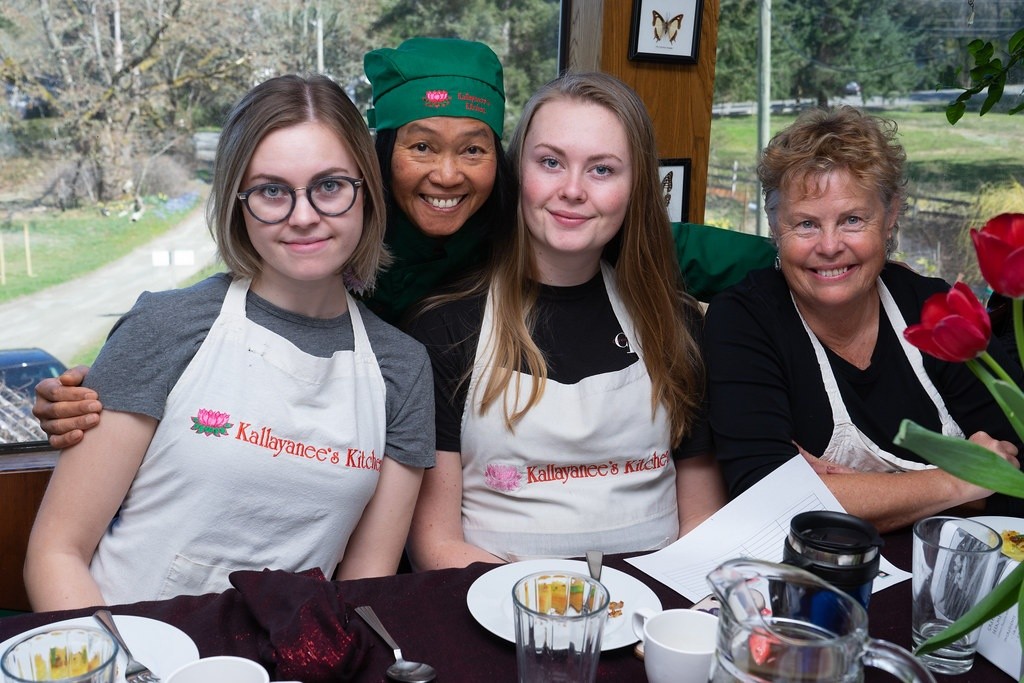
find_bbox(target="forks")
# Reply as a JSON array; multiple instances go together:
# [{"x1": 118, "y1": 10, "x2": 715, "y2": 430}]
[{"x1": 92, "y1": 610, "x2": 161, "y2": 683}]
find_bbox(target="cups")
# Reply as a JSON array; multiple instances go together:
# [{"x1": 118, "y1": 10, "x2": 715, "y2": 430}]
[
  {"x1": 511, "y1": 569, "x2": 611, "y2": 683},
  {"x1": 631, "y1": 606, "x2": 719, "y2": 683},
  {"x1": 910, "y1": 516, "x2": 1003, "y2": 676},
  {"x1": 0, "y1": 624, "x2": 121, "y2": 683}
]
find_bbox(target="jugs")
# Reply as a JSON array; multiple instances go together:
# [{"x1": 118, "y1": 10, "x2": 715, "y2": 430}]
[{"x1": 706, "y1": 558, "x2": 937, "y2": 683}]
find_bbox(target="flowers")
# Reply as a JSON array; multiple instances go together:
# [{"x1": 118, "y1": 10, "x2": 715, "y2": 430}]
[{"x1": 892, "y1": 211, "x2": 1024, "y2": 659}]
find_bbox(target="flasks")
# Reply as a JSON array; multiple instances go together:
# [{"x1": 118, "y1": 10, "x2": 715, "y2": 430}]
[{"x1": 780, "y1": 510, "x2": 886, "y2": 673}]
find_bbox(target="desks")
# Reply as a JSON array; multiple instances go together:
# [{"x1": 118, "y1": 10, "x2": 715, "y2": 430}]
[{"x1": 0, "y1": 545, "x2": 1023, "y2": 683}]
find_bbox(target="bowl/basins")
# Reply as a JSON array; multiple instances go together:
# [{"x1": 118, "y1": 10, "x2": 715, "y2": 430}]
[{"x1": 164, "y1": 655, "x2": 270, "y2": 683}]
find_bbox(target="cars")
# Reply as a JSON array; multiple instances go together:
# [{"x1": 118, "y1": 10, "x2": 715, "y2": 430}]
[{"x1": 0, "y1": 347, "x2": 68, "y2": 430}]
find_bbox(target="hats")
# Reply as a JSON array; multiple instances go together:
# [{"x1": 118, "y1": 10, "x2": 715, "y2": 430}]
[{"x1": 363, "y1": 36, "x2": 506, "y2": 140}]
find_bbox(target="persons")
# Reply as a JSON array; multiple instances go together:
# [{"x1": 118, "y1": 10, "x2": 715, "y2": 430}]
[
  {"x1": 22, "y1": 74, "x2": 437, "y2": 611},
  {"x1": 31, "y1": 37, "x2": 779, "y2": 448},
  {"x1": 703, "y1": 107, "x2": 1024, "y2": 532},
  {"x1": 399, "y1": 68, "x2": 726, "y2": 568}
]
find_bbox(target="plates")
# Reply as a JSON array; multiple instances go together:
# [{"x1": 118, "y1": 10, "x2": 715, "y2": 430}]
[
  {"x1": 0, "y1": 613, "x2": 201, "y2": 683},
  {"x1": 966, "y1": 516, "x2": 1024, "y2": 562},
  {"x1": 466, "y1": 560, "x2": 664, "y2": 653}
]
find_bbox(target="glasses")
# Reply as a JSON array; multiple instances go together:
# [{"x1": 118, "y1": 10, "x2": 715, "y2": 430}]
[{"x1": 237, "y1": 176, "x2": 364, "y2": 223}]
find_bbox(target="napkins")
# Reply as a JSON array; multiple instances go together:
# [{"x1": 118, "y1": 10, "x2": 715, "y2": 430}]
[{"x1": 226, "y1": 564, "x2": 375, "y2": 683}]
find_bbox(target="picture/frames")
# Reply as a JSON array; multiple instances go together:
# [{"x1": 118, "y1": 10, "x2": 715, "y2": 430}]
[
  {"x1": 628, "y1": 0, "x2": 703, "y2": 64},
  {"x1": 658, "y1": 158, "x2": 692, "y2": 222}
]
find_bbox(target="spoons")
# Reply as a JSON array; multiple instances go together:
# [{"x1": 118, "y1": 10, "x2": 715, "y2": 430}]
[{"x1": 354, "y1": 605, "x2": 437, "y2": 683}]
